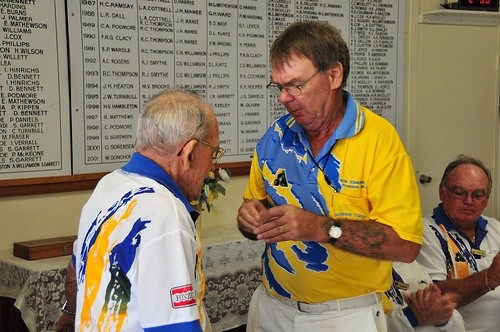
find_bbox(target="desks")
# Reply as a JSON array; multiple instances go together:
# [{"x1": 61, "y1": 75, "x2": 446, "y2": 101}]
[{"x1": 0, "y1": 175, "x2": 267, "y2": 332}]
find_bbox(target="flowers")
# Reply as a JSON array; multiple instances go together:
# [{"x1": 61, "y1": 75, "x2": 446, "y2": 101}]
[{"x1": 189, "y1": 167, "x2": 232, "y2": 214}]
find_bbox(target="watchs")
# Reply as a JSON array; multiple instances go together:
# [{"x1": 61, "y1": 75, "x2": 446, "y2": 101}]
[
  {"x1": 328, "y1": 219, "x2": 342, "y2": 244},
  {"x1": 62, "y1": 300, "x2": 75, "y2": 314}
]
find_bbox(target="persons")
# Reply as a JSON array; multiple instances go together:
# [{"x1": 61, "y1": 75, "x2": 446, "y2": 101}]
[
  {"x1": 237, "y1": 21, "x2": 423, "y2": 331},
  {"x1": 384, "y1": 157, "x2": 500, "y2": 331},
  {"x1": 56, "y1": 89, "x2": 226, "y2": 332}
]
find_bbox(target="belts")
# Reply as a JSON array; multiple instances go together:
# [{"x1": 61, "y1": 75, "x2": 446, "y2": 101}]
[{"x1": 263, "y1": 285, "x2": 383, "y2": 314}]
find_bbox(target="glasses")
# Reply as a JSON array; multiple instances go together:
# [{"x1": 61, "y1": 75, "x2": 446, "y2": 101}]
[
  {"x1": 177, "y1": 139, "x2": 225, "y2": 166},
  {"x1": 443, "y1": 182, "x2": 488, "y2": 202},
  {"x1": 266, "y1": 60, "x2": 344, "y2": 97}
]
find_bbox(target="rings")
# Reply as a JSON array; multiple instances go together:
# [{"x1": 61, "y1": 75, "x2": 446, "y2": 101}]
[{"x1": 280, "y1": 226, "x2": 284, "y2": 233}]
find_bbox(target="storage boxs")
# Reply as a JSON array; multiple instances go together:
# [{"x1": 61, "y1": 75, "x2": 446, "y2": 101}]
[{"x1": 14, "y1": 235, "x2": 77, "y2": 260}]
